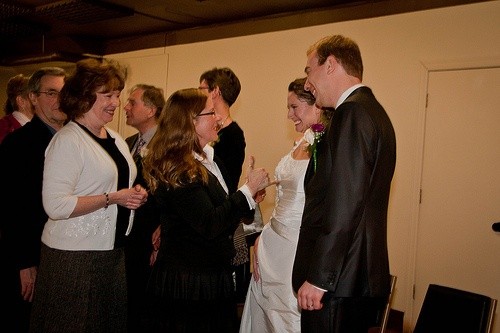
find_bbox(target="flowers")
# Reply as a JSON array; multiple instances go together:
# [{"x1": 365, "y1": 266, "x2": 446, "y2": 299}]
[
  {"x1": 207, "y1": 133, "x2": 224, "y2": 147},
  {"x1": 302, "y1": 124, "x2": 324, "y2": 177}
]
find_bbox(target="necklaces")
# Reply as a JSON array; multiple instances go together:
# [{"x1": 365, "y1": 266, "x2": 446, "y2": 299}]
[{"x1": 216, "y1": 114, "x2": 230, "y2": 131}]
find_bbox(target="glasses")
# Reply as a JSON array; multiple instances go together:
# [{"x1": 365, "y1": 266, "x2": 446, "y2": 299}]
[
  {"x1": 198, "y1": 86, "x2": 209, "y2": 91},
  {"x1": 36, "y1": 90, "x2": 59, "y2": 97},
  {"x1": 197, "y1": 109, "x2": 218, "y2": 117}
]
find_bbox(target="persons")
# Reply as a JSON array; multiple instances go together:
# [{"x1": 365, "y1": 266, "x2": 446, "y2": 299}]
[
  {"x1": 0, "y1": 73, "x2": 35, "y2": 144},
  {"x1": 124, "y1": 67, "x2": 246, "y2": 333},
  {"x1": 239, "y1": 77, "x2": 335, "y2": 333},
  {"x1": 141, "y1": 88, "x2": 278, "y2": 333},
  {"x1": 28, "y1": 57, "x2": 148, "y2": 333},
  {"x1": 291, "y1": 34, "x2": 396, "y2": 333},
  {"x1": 0, "y1": 67, "x2": 68, "y2": 333}
]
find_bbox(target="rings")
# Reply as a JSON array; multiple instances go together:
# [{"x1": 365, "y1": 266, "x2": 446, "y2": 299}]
[
  {"x1": 307, "y1": 304, "x2": 313, "y2": 307},
  {"x1": 248, "y1": 165, "x2": 254, "y2": 168}
]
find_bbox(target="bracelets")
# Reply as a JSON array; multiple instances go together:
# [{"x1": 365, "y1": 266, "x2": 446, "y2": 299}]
[{"x1": 104, "y1": 193, "x2": 109, "y2": 208}]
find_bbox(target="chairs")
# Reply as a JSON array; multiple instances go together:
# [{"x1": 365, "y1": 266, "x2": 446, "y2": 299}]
[{"x1": 413, "y1": 284, "x2": 497, "y2": 333}]
